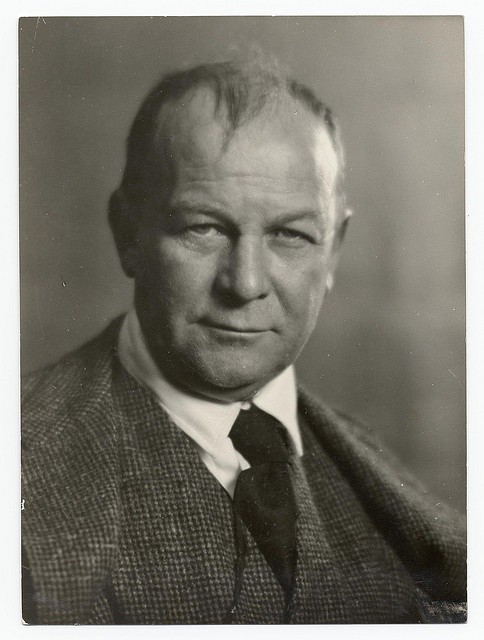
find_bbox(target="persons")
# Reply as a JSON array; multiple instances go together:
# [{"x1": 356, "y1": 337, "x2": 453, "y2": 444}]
[{"x1": 22, "y1": 60, "x2": 467, "y2": 623}]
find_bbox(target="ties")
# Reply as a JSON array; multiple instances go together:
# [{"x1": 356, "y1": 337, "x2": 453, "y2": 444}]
[{"x1": 226, "y1": 405, "x2": 297, "y2": 603}]
